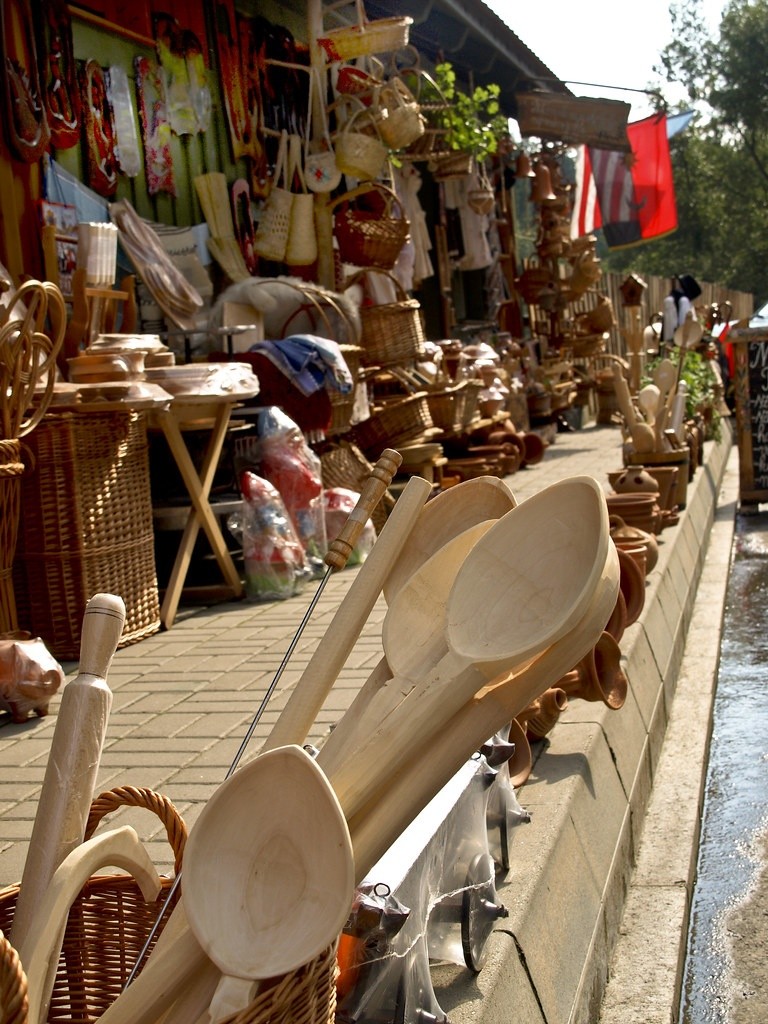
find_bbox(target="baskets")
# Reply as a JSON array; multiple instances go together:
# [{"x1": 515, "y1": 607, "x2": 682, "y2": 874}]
[
  {"x1": 255, "y1": 0, "x2": 631, "y2": 534},
  {"x1": 0, "y1": 408, "x2": 160, "y2": 660},
  {"x1": 0, "y1": 783, "x2": 340, "y2": 1023}
]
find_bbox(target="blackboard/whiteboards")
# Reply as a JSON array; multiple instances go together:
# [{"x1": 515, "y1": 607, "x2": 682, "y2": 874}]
[{"x1": 729, "y1": 323, "x2": 768, "y2": 502}]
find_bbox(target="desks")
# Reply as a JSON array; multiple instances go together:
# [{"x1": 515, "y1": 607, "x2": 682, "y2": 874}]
[{"x1": 37, "y1": 389, "x2": 248, "y2": 631}]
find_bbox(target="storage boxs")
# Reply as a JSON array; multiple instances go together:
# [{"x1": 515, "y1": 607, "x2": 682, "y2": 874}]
[
  {"x1": 263, "y1": 12, "x2": 706, "y2": 585},
  {"x1": 18, "y1": 409, "x2": 162, "y2": 664}
]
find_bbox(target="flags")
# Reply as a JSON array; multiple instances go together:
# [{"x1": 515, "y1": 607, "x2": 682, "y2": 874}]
[
  {"x1": 568, "y1": 140, "x2": 638, "y2": 244},
  {"x1": 585, "y1": 108, "x2": 679, "y2": 254}
]
